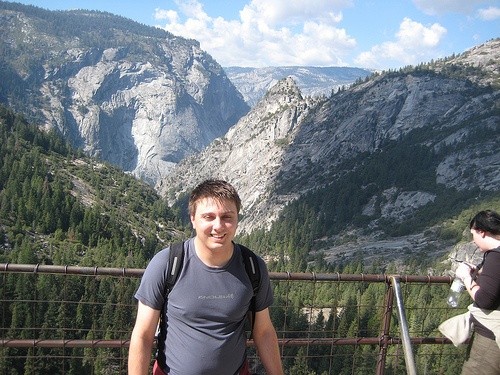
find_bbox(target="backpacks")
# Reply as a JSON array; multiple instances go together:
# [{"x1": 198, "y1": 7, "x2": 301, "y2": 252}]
[{"x1": 154, "y1": 240, "x2": 261, "y2": 375}]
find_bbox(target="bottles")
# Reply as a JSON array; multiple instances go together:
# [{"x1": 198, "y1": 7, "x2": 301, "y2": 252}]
[{"x1": 447, "y1": 277, "x2": 467, "y2": 309}]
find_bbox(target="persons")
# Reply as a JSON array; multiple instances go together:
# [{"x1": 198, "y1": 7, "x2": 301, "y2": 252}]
[
  {"x1": 455, "y1": 209, "x2": 500, "y2": 375},
  {"x1": 128, "y1": 180, "x2": 283, "y2": 375}
]
